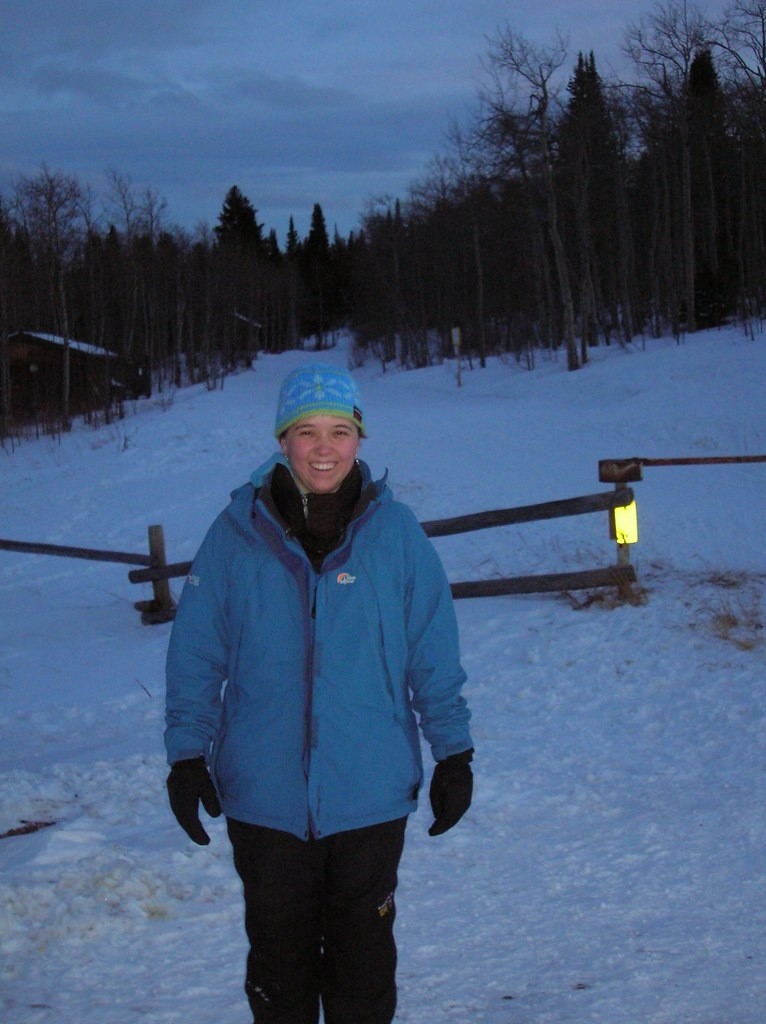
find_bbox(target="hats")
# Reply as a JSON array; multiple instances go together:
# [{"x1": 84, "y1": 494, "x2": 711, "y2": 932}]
[{"x1": 275, "y1": 362, "x2": 367, "y2": 437}]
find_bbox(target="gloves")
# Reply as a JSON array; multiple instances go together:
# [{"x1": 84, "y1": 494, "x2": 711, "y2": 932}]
[
  {"x1": 428, "y1": 749, "x2": 481, "y2": 836},
  {"x1": 166, "y1": 756, "x2": 221, "y2": 846}
]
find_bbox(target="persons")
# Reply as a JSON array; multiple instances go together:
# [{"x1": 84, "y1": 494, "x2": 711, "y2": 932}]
[{"x1": 164, "y1": 363, "x2": 474, "y2": 1022}]
